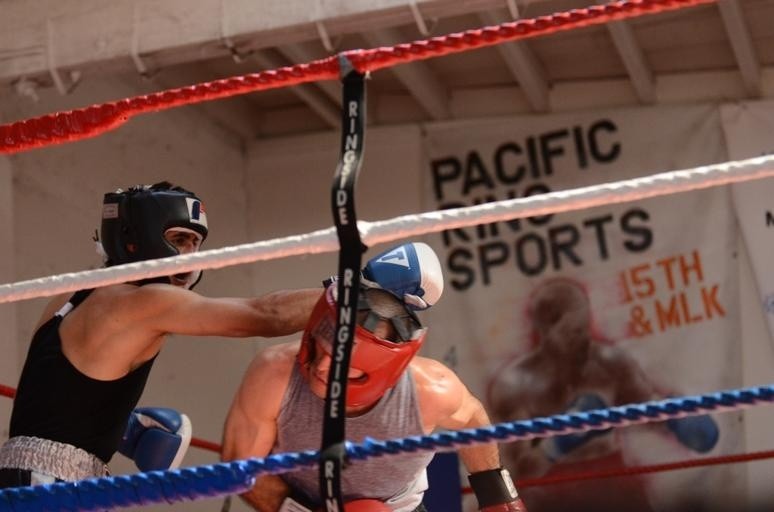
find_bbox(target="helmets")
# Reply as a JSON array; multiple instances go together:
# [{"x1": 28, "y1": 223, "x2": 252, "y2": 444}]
[
  {"x1": 100, "y1": 180, "x2": 207, "y2": 291},
  {"x1": 296, "y1": 279, "x2": 428, "y2": 408}
]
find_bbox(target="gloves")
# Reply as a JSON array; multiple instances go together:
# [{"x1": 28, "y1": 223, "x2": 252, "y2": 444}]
[
  {"x1": 538, "y1": 393, "x2": 611, "y2": 461},
  {"x1": 361, "y1": 241, "x2": 443, "y2": 311},
  {"x1": 664, "y1": 392, "x2": 718, "y2": 452},
  {"x1": 467, "y1": 465, "x2": 527, "y2": 512},
  {"x1": 118, "y1": 406, "x2": 192, "y2": 472},
  {"x1": 277, "y1": 485, "x2": 393, "y2": 512}
]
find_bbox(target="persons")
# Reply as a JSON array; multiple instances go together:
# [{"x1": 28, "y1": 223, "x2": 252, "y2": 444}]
[
  {"x1": 219, "y1": 274, "x2": 528, "y2": 511},
  {"x1": 2, "y1": 179, "x2": 447, "y2": 511},
  {"x1": 487, "y1": 275, "x2": 720, "y2": 512}
]
